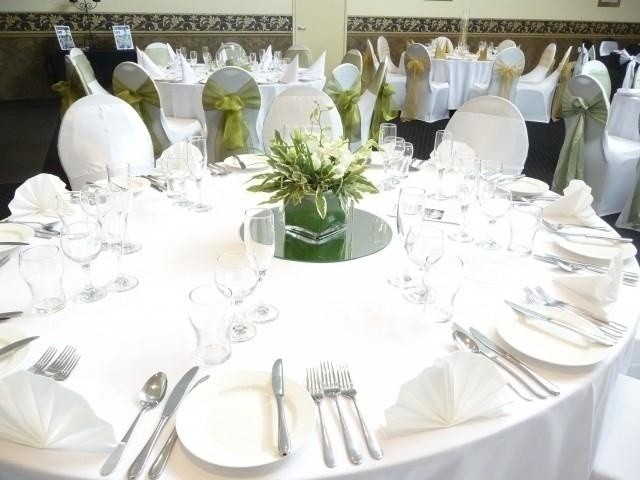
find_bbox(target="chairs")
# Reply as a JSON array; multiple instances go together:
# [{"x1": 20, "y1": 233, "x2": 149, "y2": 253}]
[
  {"x1": 341, "y1": 49, "x2": 364, "y2": 77},
  {"x1": 580, "y1": 59, "x2": 611, "y2": 106},
  {"x1": 64, "y1": 48, "x2": 112, "y2": 100},
  {"x1": 322, "y1": 62, "x2": 361, "y2": 154},
  {"x1": 60, "y1": 91, "x2": 156, "y2": 176},
  {"x1": 355, "y1": 55, "x2": 390, "y2": 148},
  {"x1": 373, "y1": 37, "x2": 400, "y2": 74},
  {"x1": 219, "y1": 41, "x2": 251, "y2": 64},
  {"x1": 551, "y1": 74, "x2": 640, "y2": 232},
  {"x1": 494, "y1": 39, "x2": 517, "y2": 54},
  {"x1": 570, "y1": 41, "x2": 598, "y2": 77},
  {"x1": 360, "y1": 38, "x2": 409, "y2": 112},
  {"x1": 599, "y1": 40, "x2": 618, "y2": 57},
  {"x1": 432, "y1": 37, "x2": 454, "y2": 53},
  {"x1": 514, "y1": 45, "x2": 574, "y2": 124},
  {"x1": 262, "y1": 83, "x2": 345, "y2": 156},
  {"x1": 440, "y1": 95, "x2": 528, "y2": 174},
  {"x1": 144, "y1": 42, "x2": 174, "y2": 67},
  {"x1": 466, "y1": 48, "x2": 525, "y2": 107},
  {"x1": 280, "y1": 43, "x2": 314, "y2": 69},
  {"x1": 110, "y1": 62, "x2": 208, "y2": 165},
  {"x1": 399, "y1": 44, "x2": 450, "y2": 124},
  {"x1": 519, "y1": 42, "x2": 557, "y2": 83}
]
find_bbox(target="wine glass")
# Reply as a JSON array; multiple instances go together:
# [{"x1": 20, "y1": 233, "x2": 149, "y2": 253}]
[
  {"x1": 480, "y1": 184, "x2": 512, "y2": 251},
  {"x1": 215, "y1": 205, "x2": 278, "y2": 342},
  {"x1": 431, "y1": 130, "x2": 478, "y2": 243},
  {"x1": 187, "y1": 135, "x2": 213, "y2": 213},
  {"x1": 59, "y1": 182, "x2": 138, "y2": 303},
  {"x1": 387, "y1": 186, "x2": 447, "y2": 306},
  {"x1": 173, "y1": 45, "x2": 284, "y2": 75},
  {"x1": 376, "y1": 122, "x2": 404, "y2": 192}
]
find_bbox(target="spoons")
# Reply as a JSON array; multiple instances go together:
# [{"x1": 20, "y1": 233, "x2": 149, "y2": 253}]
[
  {"x1": 557, "y1": 260, "x2": 636, "y2": 286},
  {"x1": 451, "y1": 330, "x2": 545, "y2": 399},
  {"x1": 98, "y1": 370, "x2": 167, "y2": 476}
]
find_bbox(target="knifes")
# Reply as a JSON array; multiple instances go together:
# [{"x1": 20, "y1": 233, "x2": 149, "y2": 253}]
[
  {"x1": 270, "y1": 356, "x2": 291, "y2": 461},
  {"x1": 228, "y1": 153, "x2": 247, "y2": 168},
  {"x1": 502, "y1": 300, "x2": 614, "y2": 349},
  {"x1": 1, "y1": 310, "x2": 23, "y2": 321},
  {"x1": 127, "y1": 366, "x2": 212, "y2": 480},
  {"x1": 0, "y1": 336, "x2": 41, "y2": 357},
  {"x1": 454, "y1": 323, "x2": 562, "y2": 399}
]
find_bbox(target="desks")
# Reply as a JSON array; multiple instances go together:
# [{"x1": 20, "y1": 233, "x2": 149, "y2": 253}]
[
  {"x1": 603, "y1": 87, "x2": 639, "y2": 140},
  {"x1": 397, "y1": 51, "x2": 496, "y2": 114},
  {"x1": 137, "y1": 61, "x2": 327, "y2": 157}
]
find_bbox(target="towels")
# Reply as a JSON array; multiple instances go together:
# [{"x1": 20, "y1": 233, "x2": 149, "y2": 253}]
[{"x1": 5, "y1": 173, "x2": 73, "y2": 222}]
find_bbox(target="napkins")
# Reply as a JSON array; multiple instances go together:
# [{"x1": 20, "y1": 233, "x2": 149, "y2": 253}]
[
  {"x1": 153, "y1": 138, "x2": 203, "y2": 176},
  {"x1": 539, "y1": 178, "x2": 595, "y2": 225},
  {"x1": 3, "y1": 171, "x2": 78, "y2": 220},
  {"x1": 278, "y1": 51, "x2": 299, "y2": 83},
  {"x1": 555, "y1": 251, "x2": 629, "y2": 302},
  {"x1": 136, "y1": 47, "x2": 164, "y2": 80},
  {"x1": 428, "y1": 139, "x2": 479, "y2": 168},
  {"x1": 259, "y1": 42, "x2": 274, "y2": 69},
  {"x1": 376, "y1": 346, "x2": 513, "y2": 439},
  {"x1": 303, "y1": 48, "x2": 328, "y2": 78}
]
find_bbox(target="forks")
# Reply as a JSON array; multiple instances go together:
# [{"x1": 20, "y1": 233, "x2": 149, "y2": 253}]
[
  {"x1": 27, "y1": 344, "x2": 82, "y2": 381},
  {"x1": 520, "y1": 285, "x2": 628, "y2": 341},
  {"x1": 305, "y1": 361, "x2": 387, "y2": 470}
]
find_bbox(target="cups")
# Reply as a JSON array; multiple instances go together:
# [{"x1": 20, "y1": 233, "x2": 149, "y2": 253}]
[
  {"x1": 397, "y1": 142, "x2": 413, "y2": 179},
  {"x1": 18, "y1": 245, "x2": 67, "y2": 315},
  {"x1": 106, "y1": 161, "x2": 133, "y2": 194},
  {"x1": 186, "y1": 283, "x2": 237, "y2": 366},
  {"x1": 506, "y1": 202, "x2": 544, "y2": 255}
]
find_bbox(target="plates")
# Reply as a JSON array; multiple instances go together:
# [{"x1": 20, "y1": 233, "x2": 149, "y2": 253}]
[
  {"x1": 223, "y1": 153, "x2": 271, "y2": 169},
  {"x1": 174, "y1": 370, "x2": 319, "y2": 470},
  {"x1": 93, "y1": 177, "x2": 151, "y2": 196},
  {"x1": 495, "y1": 176, "x2": 549, "y2": 195},
  {"x1": 555, "y1": 225, "x2": 637, "y2": 261},
  {"x1": 0, "y1": 222, "x2": 35, "y2": 254},
  {"x1": 495, "y1": 298, "x2": 629, "y2": 368}
]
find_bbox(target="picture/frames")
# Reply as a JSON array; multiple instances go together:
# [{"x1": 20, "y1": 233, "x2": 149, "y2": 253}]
[
  {"x1": 112, "y1": 25, "x2": 135, "y2": 50},
  {"x1": 54, "y1": 26, "x2": 76, "y2": 51}
]
